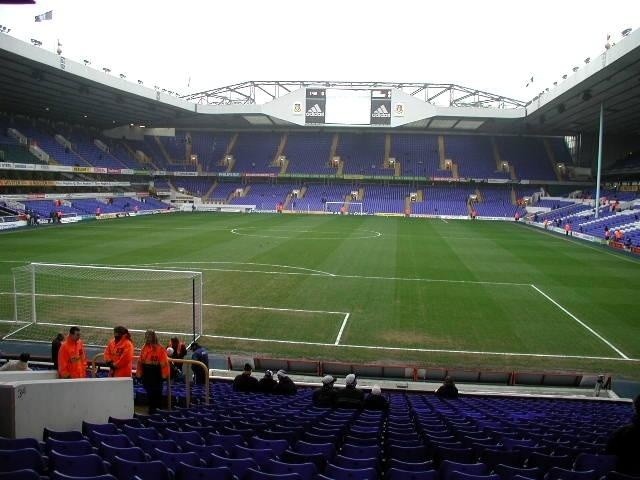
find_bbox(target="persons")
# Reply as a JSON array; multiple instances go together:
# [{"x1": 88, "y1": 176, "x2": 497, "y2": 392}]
[
  {"x1": 311, "y1": 372, "x2": 387, "y2": 411},
  {"x1": 565, "y1": 223, "x2": 570, "y2": 235},
  {"x1": 278, "y1": 202, "x2": 283, "y2": 213},
  {"x1": 233, "y1": 363, "x2": 297, "y2": 396},
  {"x1": 570, "y1": 220, "x2": 573, "y2": 237},
  {"x1": 50, "y1": 209, "x2": 63, "y2": 225},
  {"x1": 604, "y1": 226, "x2": 632, "y2": 257},
  {"x1": 599, "y1": 196, "x2": 619, "y2": 213},
  {"x1": 108, "y1": 196, "x2": 113, "y2": 205},
  {"x1": 27, "y1": 209, "x2": 41, "y2": 226},
  {"x1": 515, "y1": 212, "x2": 561, "y2": 230},
  {"x1": 167, "y1": 203, "x2": 170, "y2": 210},
  {"x1": 434, "y1": 375, "x2": 459, "y2": 399},
  {"x1": 605, "y1": 393, "x2": 640, "y2": 456},
  {"x1": 52, "y1": 324, "x2": 209, "y2": 415},
  {"x1": 328, "y1": 205, "x2": 332, "y2": 212},
  {"x1": 96, "y1": 205, "x2": 101, "y2": 216},
  {"x1": 472, "y1": 209, "x2": 475, "y2": 219},
  {"x1": 134, "y1": 205, "x2": 137, "y2": 213},
  {"x1": 593, "y1": 376, "x2": 604, "y2": 396},
  {"x1": 0, "y1": 351, "x2": 34, "y2": 371}
]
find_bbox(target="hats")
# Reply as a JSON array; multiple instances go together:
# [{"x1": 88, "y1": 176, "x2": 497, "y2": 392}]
[
  {"x1": 265, "y1": 370, "x2": 274, "y2": 377},
  {"x1": 322, "y1": 375, "x2": 337, "y2": 385},
  {"x1": 345, "y1": 373, "x2": 358, "y2": 385},
  {"x1": 244, "y1": 363, "x2": 253, "y2": 371},
  {"x1": 277, "y1": 370, "x2": 288, "y2": 378},
  {"x1": 371, "y1": 384, "x2": 382, "y2": 396},
  {"x1": 189, "y1": 342, "x2": 200, "y2": 350}
]
